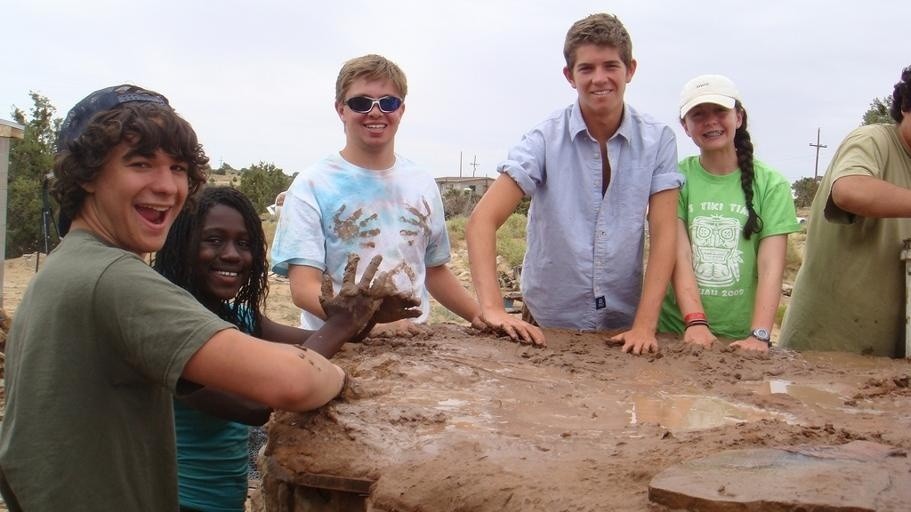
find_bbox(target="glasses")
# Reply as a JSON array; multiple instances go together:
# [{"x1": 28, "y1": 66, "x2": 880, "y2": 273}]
[{"x1": 343, "y1": 92, "x2": 403, "y2": 116}]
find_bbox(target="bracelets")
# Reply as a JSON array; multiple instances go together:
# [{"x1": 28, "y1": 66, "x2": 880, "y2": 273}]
[{"x1": 683, "y1": 312, "x2": 709, "y2": 331}]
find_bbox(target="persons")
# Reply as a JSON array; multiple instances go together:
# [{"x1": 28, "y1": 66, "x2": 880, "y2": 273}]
[
  {"x1": 465, "y1": 14, "x2": 685, "y2": 356},
  {"x1": 0, "y1": 85, "x2": 345, "y2": 512},
  {"x1": 146, "y1": 186, "x2": 423, "y2": 512},
  {"x1": 779, "y1": 66, "x2": 911, "y2": 359},
  {"x1": 270, "y1": 55, "x2": 506, "y2": 338},
  {"x1": 665, "y1": 76, "x2": 800, "y2": 360}
]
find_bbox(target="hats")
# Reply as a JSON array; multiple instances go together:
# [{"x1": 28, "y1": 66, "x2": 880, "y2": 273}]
[
  {"x1": 673, "y1": 71, "x2": 745, "y2": 121},
  {"x1": 51, "y1": 81, "x2": 170, "y2": 154}
]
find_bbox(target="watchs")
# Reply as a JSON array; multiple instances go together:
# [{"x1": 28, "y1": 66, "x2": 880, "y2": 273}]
[{"x1": 747, "y1": 328, "x2": 771, "y2": 341}]
